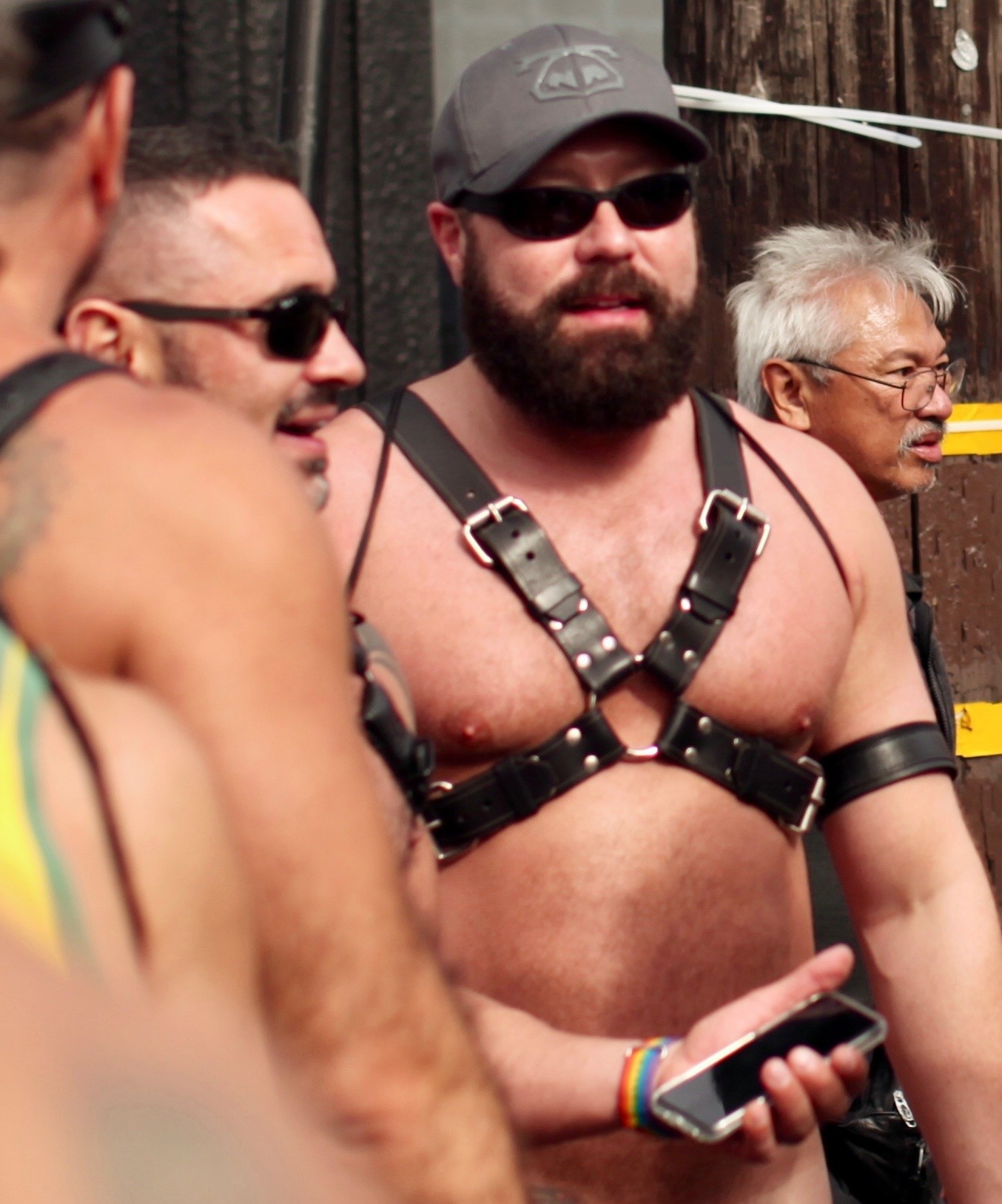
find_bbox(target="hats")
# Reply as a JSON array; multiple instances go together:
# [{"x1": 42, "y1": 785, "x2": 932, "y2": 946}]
[{"x1": 430, "y1": 24, "x2": 713, "y2": 206}]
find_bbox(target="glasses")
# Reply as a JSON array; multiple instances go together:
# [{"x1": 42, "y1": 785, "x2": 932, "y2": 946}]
[
  {"x1": 113, "y1": 287, "x2": 358, "y2": 360},
  {"x1": 446, "y1": 171, "x2": 695, "y2": 240},
  {"x1": 789, "y1": 356, "x2": 967, "y2": 411}
]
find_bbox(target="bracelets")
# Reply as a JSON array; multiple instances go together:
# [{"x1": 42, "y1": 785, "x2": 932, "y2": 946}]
[{"x1": 618, "y1": 1033, "x2": 678, "y2": 1141}]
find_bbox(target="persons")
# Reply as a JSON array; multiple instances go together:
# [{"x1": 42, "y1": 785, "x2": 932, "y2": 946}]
[
  {"x1": 313, "y1": 24, "x2": 1001, "y2": 1203},
  {"x1": 53, "y1": 129, "x2": 364, "y2": 518},
  {"x1": 0, "y1": 929, "x2": 387, "y2": 1204},
  {"x1": 6, "y1": 616, "x2": 267, "y2": 1056},
  {"x1": 3, "y1": 0, "x2": 534, "y2": 1194},
  {"x1": 720, "y1": 221, "x2": 968, "y2": 1201}
]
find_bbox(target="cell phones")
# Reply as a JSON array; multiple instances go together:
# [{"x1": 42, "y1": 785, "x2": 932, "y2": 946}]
[{"x1": 649, "y1": 986, "x2": 888, "y2": 1144}]
